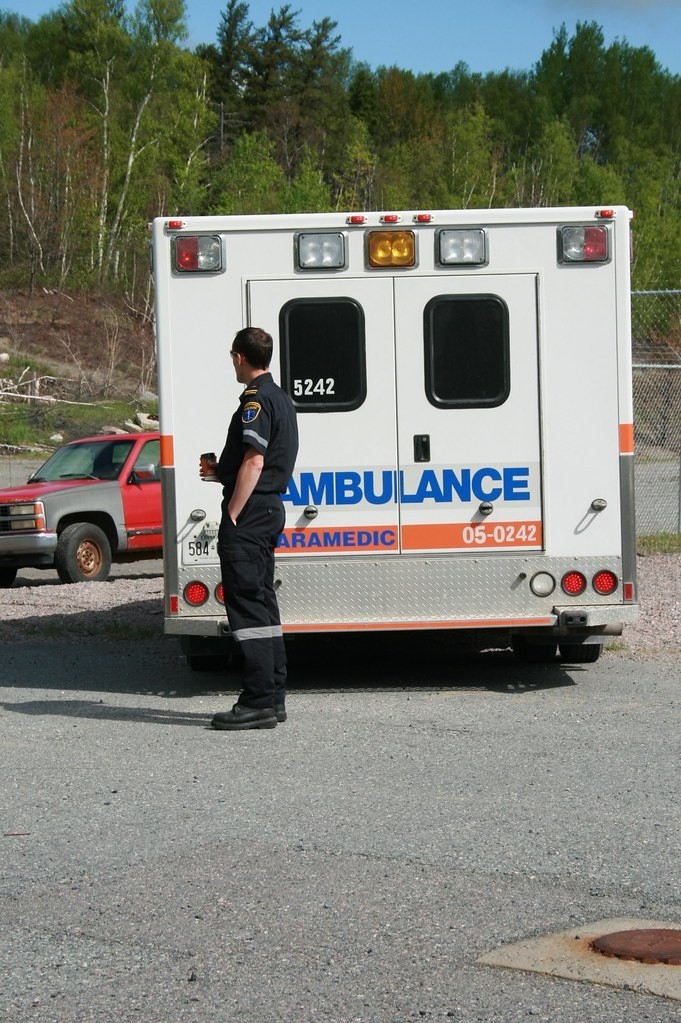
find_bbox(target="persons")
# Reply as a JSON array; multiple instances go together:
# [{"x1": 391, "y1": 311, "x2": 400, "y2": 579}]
[{"x1": 199, "y1": 327, "x2": 300, "y2": 731}]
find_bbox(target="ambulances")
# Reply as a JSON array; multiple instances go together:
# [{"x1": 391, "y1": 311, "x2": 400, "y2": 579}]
[{"x1": 149, "y1": 204, "x2": 642, "y2": 665}]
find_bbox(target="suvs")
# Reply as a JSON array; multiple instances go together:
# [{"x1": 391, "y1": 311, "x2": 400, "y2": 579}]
[{"x1": 0, "y1": 432, "x2": 163, "y2": 588}]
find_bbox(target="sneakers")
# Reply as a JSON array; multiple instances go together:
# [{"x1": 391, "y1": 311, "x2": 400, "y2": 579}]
[{"x1": 210, "y1": 701, "x2": 287, "y2": 731}]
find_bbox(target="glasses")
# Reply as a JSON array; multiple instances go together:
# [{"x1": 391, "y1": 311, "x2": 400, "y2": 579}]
[{"x1": 230, "y1": 350, "x2": 243, "y2": 358}]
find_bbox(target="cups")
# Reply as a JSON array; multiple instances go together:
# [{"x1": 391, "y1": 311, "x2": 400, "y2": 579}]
[{"x1": 200, "y1": 452, "x2": 217, "y2": 482}]
